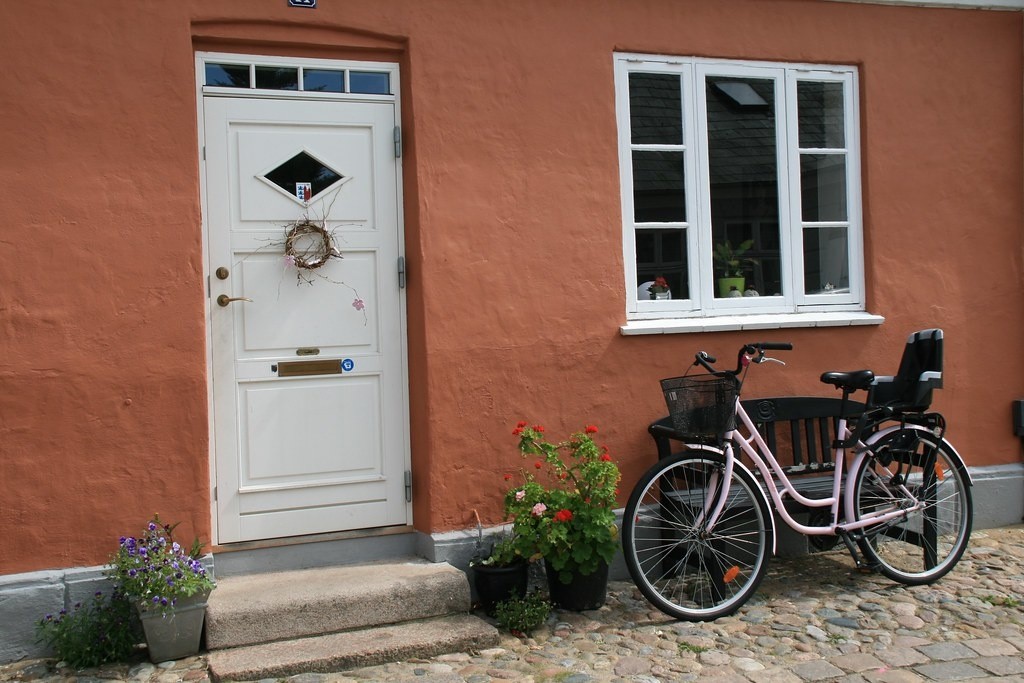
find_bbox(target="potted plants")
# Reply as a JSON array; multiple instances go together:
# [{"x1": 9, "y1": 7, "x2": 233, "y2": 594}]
[{"x1": 713, "y1": 241, "x2": 762, "y2": 297}]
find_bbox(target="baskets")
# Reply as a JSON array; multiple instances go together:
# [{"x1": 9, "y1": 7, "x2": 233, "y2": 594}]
[{"x1": 659, "y1": 373, "x2": 736, "y2": 439}]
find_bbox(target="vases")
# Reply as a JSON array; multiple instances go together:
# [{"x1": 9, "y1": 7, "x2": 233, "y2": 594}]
[
  {"x1": 136, "y1": 560, "x2": 216, "y2": 662},
  {"x1": 544, "y1": 550, "x2": 608, "y2": 610},
  {"x1": 471, "y1": 554, "x2": 530, "y2": 618},
  {"x1": 650, "y1": 293, "x2": 672, "y2": 299}
]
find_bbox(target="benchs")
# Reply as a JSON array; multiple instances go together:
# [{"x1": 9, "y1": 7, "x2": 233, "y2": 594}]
[{"x1": 647, "y1": 397, "x2": 945, "y2": 601}]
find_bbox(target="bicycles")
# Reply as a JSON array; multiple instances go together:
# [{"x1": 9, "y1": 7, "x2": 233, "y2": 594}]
[{"x1": 621, "y1": 329, "x2": 973, "y2": 621}]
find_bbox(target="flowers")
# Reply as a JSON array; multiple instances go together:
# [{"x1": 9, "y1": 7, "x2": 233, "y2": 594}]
[
  {"x1": 100, "y1": 512, "x2": 212, "y2": 614},
  {"x1": 283, "y1": 255, "x2": 296, "y2": 266},
  {"x1": 647, "y1": 277, "x2": 670, "y2": 293},
  {"x1": 353, "y1": 299, "x2": 365, "y2": 310},
  {"x1": 469, "y1": 421, "x2": 640, "y2": 584}
]
